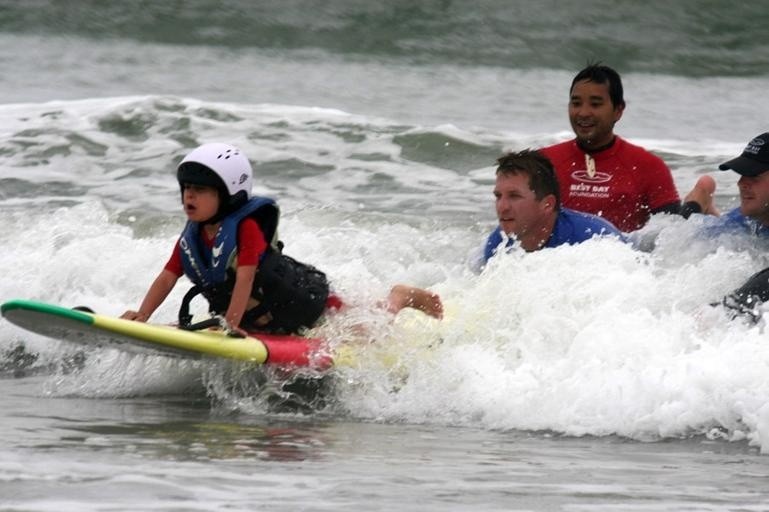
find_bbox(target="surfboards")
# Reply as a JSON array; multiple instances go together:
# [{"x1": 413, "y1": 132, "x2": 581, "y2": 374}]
[{"x1": 0, "y1": 299, "x2": 426, "y2": 372}]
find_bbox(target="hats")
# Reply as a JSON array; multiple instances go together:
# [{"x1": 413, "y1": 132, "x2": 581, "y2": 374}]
[{"x1": 718, "y1": 132, "x2": 768, "y2": 176}]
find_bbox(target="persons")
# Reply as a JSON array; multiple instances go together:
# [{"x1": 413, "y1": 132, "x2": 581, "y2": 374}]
[
  {"x1": 483, "y1": 149, "x2": 718, "y2": 270},
  {"x1": 702, "y1": 132, "x2": 769, "y2": 242},
  {"x1": 118, "y1": 143, "x2": 444, "y2": 339},
  {"x1": 538, "y1": 66, "x2": 682, "y2": 233}
]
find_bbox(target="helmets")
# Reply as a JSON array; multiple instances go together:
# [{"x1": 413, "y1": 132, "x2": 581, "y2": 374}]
[{"x1": 177, "y1": 143, "x2": 253, "y2": 203}]
